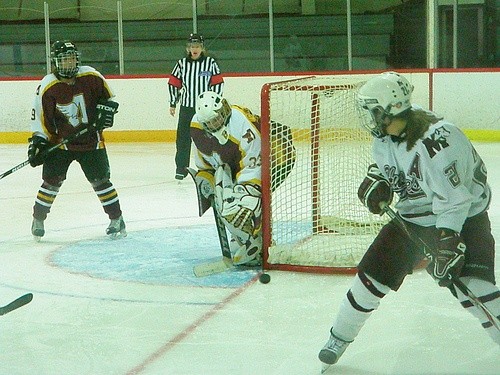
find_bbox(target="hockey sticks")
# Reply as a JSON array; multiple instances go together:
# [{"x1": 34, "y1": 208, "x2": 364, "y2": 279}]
[
  {"x1": 0, "y1": 293, "x2": 34, "y2": 316},
  {"x1": 380, "y1": 203, "x2": 500, "y2": 332},
  {"x1": 0, "y1": 122, "x2": 98, "y2": 180},
  {"x1": 192, "y1": 196, "x2": 233, "y2": 278}
]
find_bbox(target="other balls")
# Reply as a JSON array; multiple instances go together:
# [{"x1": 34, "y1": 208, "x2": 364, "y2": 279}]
[{"x1": 258, "y1": 274, "x2": 271, "y2": 284}]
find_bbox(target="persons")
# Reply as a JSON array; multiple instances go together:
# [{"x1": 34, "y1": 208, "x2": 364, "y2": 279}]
[
  {"x1": 28, "y1": 40, "x2": 127, "y2": 238},
  {"x1": 168, "y1": 35, "x2": 224, "y2": 184},
  {"x1": 191, "y1": 92, "x2": 296, "y2": 264},
  {"x1": 318, "y1": 71, "x2": 500, "y2": 375}
]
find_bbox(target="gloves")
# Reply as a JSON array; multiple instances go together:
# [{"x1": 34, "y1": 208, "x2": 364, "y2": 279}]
[
  {"x1": 28, "y1": 136, "x2": 54, "y2": 168},
  {"x1": 425, "y1": 234, "x2": 466, "y2": 289},
  {"x1": 93, "y1": 98, "x2": 119, "y2": 132},
  {"x1": 357, "y1": 166, "x2": 393, "y2": 215}
]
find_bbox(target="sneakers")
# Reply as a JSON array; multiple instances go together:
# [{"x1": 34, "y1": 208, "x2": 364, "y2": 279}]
[
  {"x1": 318, "y1": 331, "x2": 350, "y2": 375},
  {"x1": 175, "y1": 172, "x2": 185, "y2": 184},
  {"x1": 30, "y1": 219, "x2": 45, "y2": 242},
  {"x1": 106, "y1": 216, "x2": 127, "y2": 240}
]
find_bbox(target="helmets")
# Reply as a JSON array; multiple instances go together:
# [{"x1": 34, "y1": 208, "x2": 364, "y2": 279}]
[
  {"x1": 357, "y1": 71, "x2": 415, "y2": 116},
  {"x1": 187, "y1": 33, "x2": 202, "y2": 44},
  {"x1": 195, "y1": 91, "x2": 223, "y2": 123},
  {"x1": 50, "y1": 39, "x2": 77, "y2": 66}
]
